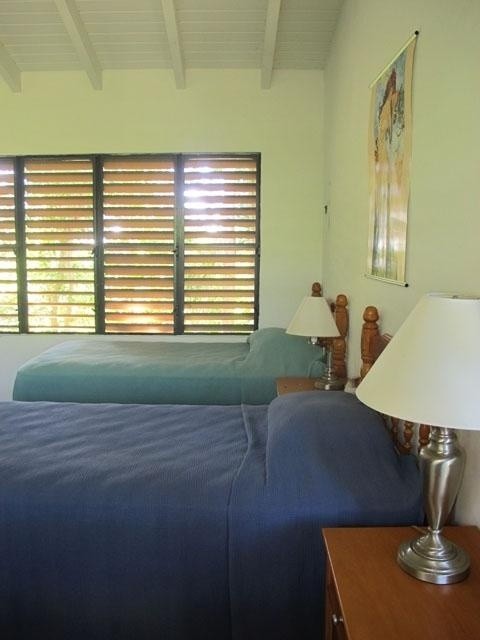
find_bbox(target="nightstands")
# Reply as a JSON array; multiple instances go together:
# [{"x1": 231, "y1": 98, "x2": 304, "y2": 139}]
[
  {"x1": 273, "y1": 374, "x2": 328, "y2": 397},
  {"x1": 320, "y1": 525, "x2": 480, "y2": 640}
]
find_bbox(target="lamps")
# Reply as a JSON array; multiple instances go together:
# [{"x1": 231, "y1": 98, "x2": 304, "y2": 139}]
[
  {"x1": 284, "y1": 294, "x2": 350, "y2": 394},
  {"x1": 355, "y1": 293, "x2": 480, "y2": 588}
]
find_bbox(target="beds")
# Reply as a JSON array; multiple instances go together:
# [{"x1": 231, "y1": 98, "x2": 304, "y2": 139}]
[
  {"x1": 9, "y1": 324, "x2": 327, "y2": 404},
  {"x1": 0, "y1": 389, "x2": 430, "y2": 640}
]
